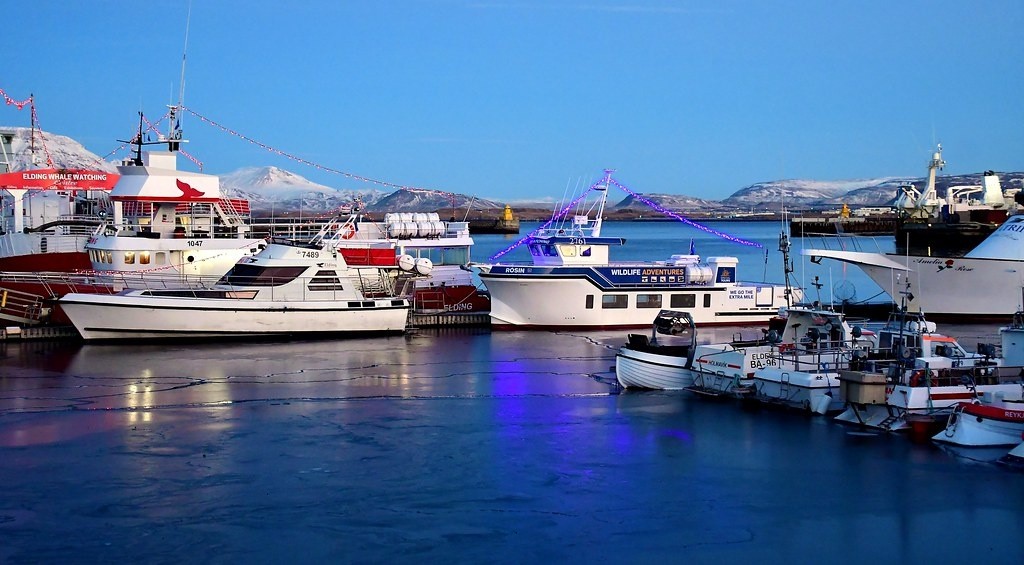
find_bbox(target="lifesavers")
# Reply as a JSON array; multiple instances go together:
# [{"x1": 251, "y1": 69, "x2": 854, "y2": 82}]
[
  {"x1": 778, "y1": 342, "x2": 797, "y2": 357},
  {"x1": 908, "y1": 369, "x2": 938, "y2": 390},
  {"x1": 338, "y1": 224, "x2": 355, "y2": 239}
]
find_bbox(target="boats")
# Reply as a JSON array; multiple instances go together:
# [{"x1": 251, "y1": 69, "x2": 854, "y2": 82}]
[
  {"x1": 470, "y1": 169, "x2": 805, "y2": 329},
  {"x1": 57, "y1": 214, "x2": 411, "y2": 340},
  {"x1": 0, "y1": 130, "x2": 492, "y2": 340},
  {"x1": 615, "y1": 306, "x2": 1024, "y2": 467},
  {"x1": 800, "y1": 214, "x2": 1024, "y2": 325}
]
